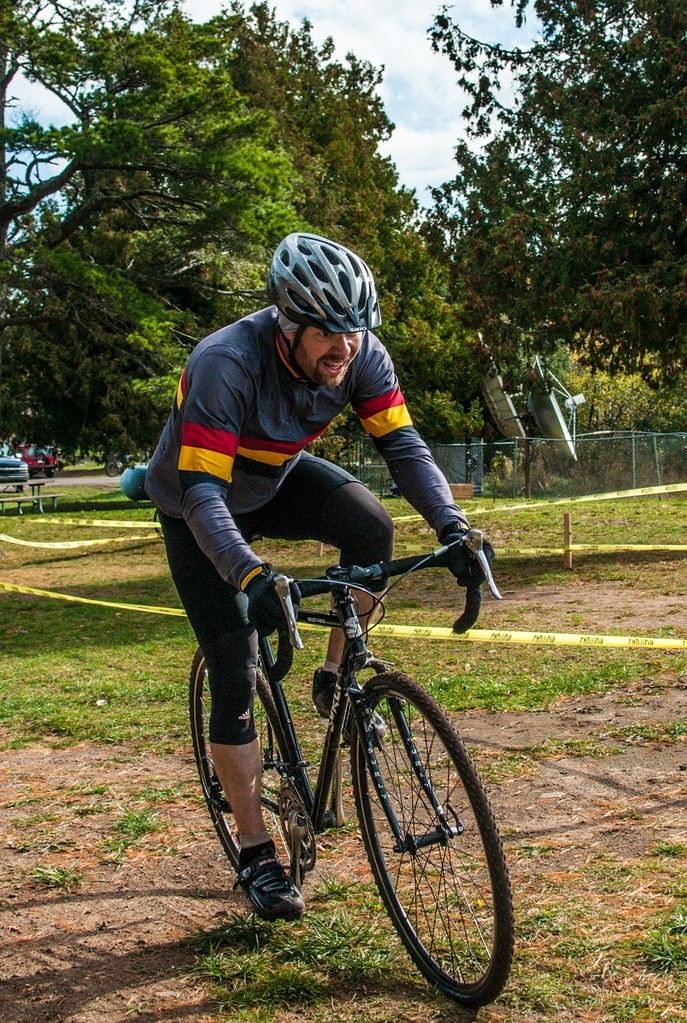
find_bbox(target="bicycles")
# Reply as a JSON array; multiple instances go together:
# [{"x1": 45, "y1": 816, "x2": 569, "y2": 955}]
[{"x1": 187, "y1": 529, "x2": 516, "y2": 1008}]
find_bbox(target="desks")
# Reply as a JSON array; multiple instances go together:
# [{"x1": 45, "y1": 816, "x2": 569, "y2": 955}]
[{"x1": 0, "y1": 480, "x2": 55, "y2": 513}]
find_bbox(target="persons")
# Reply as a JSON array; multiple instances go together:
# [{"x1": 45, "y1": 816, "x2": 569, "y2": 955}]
[{"x1": 146, "y1": 234, "x2": 494, "y2": 918}]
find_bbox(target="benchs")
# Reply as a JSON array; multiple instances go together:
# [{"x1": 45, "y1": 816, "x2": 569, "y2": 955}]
[
  {"x1": 36, "y1": 493, "x2": 67, "y2": 513},
  {"x1": 0, "y1": 496, "x2": 35, "y2": 516}
]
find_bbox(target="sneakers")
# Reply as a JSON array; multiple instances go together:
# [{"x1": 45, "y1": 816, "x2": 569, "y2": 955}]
[
  {"x1": 232, "y1": 840, "x2": 304, "y2": 922},
  {"x1": 316, "y1": 665, "x2": 384, "y2": 743}
]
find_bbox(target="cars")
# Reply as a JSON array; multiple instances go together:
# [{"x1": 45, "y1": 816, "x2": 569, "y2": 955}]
[
  {"x1": 12, "y1": 444, "x2": 66, "y2": 478},
  {"x1": 0, "y1": 440, "x2": 30, "y2": 482}
]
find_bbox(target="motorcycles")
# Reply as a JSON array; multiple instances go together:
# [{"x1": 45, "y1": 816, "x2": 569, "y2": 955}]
[{"x1": 104, "y1": 454, "x2": 137, "y2": 478}]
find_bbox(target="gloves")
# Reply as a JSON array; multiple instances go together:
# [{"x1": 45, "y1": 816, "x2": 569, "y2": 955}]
[
  {"x1": 240, "y1": 563, "x2": 301, "y2": 637},
  {"x1": 440, "y1": 521, "x2": 493, "y2": 587}
]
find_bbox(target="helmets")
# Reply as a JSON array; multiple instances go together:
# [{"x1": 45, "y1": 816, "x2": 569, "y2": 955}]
[{"x1": 268, "y1": 233, "x2": 382, "y2": 333}]
